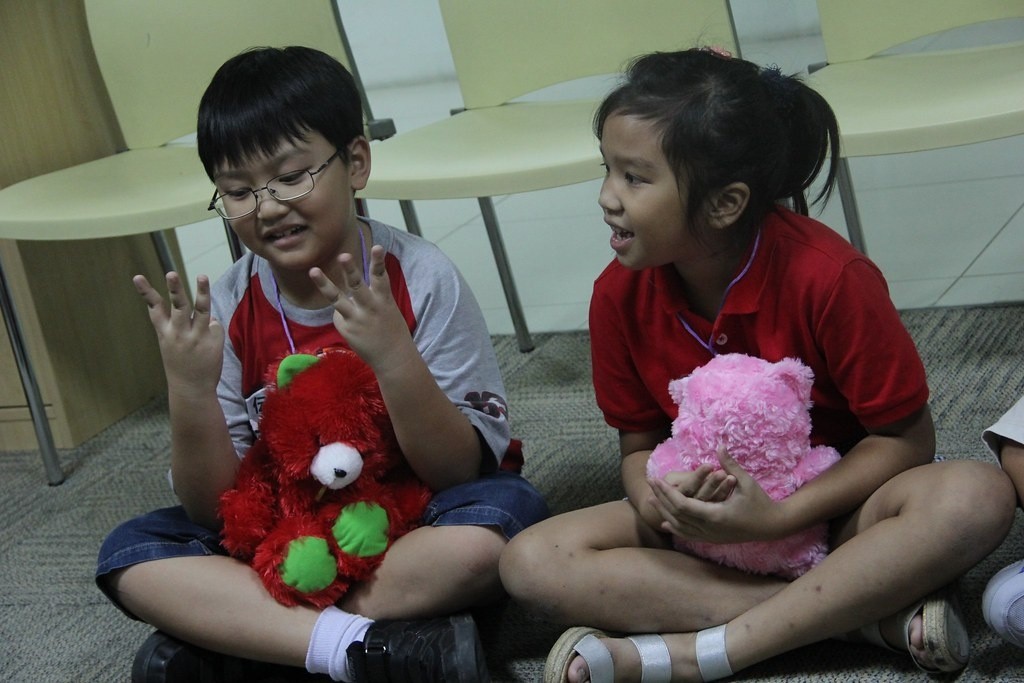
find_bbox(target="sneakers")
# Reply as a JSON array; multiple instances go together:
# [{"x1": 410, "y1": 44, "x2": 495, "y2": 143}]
[
  {"x1": 981, "y1": 558, "x2": 1023, "y2": 649},
  {"x1": 346, "y1": 610, "x2": 490, "y2": 683},
  {"x1": 131, "y1": 630, "x2": 318, "y2": 683}
]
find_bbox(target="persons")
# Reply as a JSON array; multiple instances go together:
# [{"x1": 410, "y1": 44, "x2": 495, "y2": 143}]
[
  {"x1": 980, "y1": 393, "x2": 1024, "y2": 646},
  {"x1": 96, "y1": 45, "x2": 546, "y2": 683},
  {"x1": 496, "y1": 49, "x2": 1015, "y2": 683}
]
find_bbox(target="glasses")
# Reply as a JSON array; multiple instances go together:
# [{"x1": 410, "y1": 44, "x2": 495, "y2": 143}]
[{"x1": 206, "y1": 147, "x2": 345, "y2": 220}]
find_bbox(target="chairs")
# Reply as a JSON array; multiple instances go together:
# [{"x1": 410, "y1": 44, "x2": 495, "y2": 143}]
[
  {"x1": 787, "y1": 1, "x2": 1024, "y2": 260},
  {"x1": 343, "y1": 0, "x2": 743, "y2": 354},
  {"x1": 0, "y1": 0, "x2": 423, "y2": 486}
]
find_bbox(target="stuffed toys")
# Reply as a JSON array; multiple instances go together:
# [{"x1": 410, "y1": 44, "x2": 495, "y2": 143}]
[
  {"x1": 218, "y1": 349, "x2": 434, "y2": 612},
  {"x1": 645, "y1": 357, "x2": 842, "y2": 581}
]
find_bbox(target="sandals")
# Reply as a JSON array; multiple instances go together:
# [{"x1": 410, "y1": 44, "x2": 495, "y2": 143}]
[
  {"x1": 860, "y1": 587, "x2": 971, "y2": 674},
  {"x1": 541, "y1": 625, "x2": 733, "y2": 683}
]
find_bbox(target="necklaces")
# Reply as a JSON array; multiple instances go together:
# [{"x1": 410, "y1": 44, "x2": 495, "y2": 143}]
[
  {"x1": 676, "y1": 228, "x2": 760, "y2": 357},
  {"x1": 268, "y1": 226, "x2": 368, "y2": 354}
]
